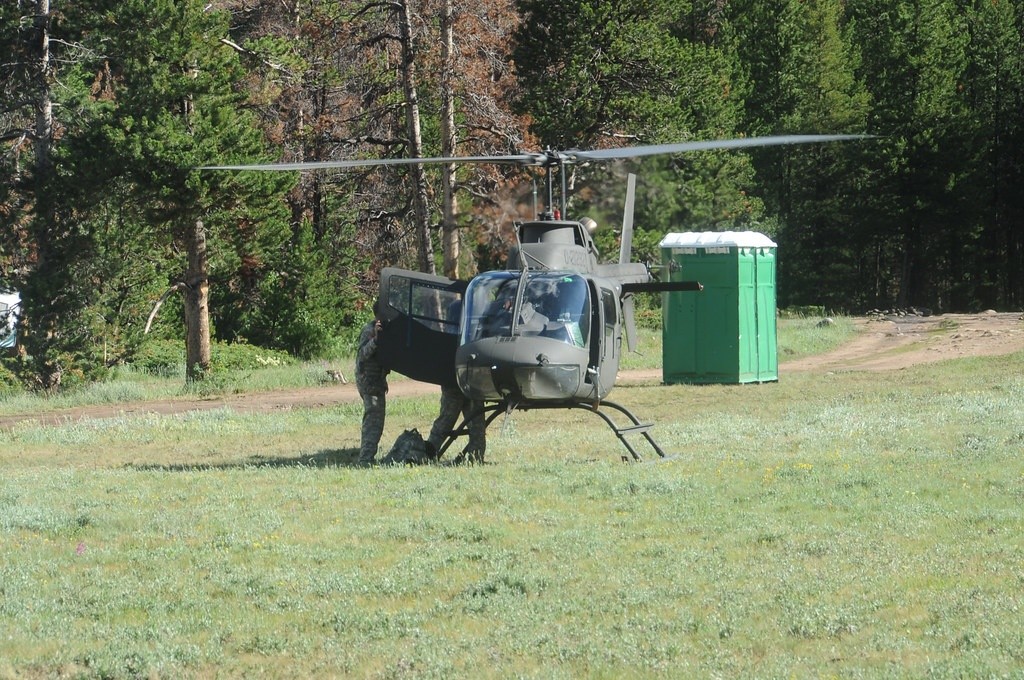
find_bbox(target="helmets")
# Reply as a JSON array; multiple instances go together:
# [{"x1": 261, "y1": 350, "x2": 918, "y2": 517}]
[{"x1": 496, "y1": 289, "x2": 516, "y2": 307}]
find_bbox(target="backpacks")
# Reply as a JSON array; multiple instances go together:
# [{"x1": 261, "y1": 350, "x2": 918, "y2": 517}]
[{"x1": 382, "y1": 428, "x2": 429, "y2": 466}]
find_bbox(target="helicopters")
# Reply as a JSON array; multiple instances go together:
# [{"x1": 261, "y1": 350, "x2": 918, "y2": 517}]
[{"x1": 195, "y1": 135, "x2": 871, "y2": 467}]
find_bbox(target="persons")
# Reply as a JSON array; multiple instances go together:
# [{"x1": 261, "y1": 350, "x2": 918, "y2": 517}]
[
  {"x1": 426, "y1": 294, "x2": 486, "y2": 466},
  {"x1": 354, "y1": 300, "x2": 390, "y2": 466}
]
